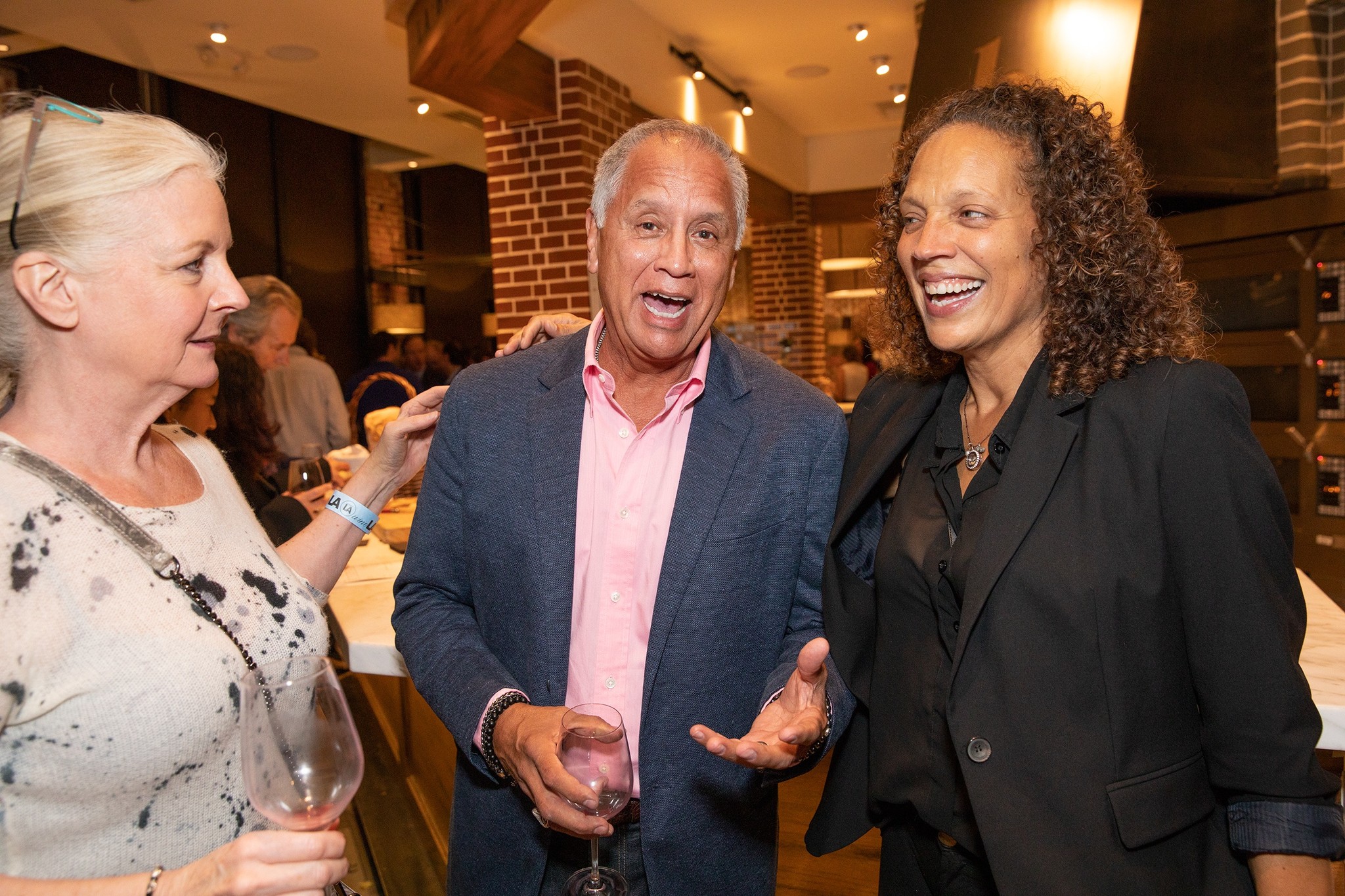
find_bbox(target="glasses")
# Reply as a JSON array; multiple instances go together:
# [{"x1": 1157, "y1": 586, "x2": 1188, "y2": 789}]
[{"x1": 9, "y1": 96, "x2": 105, "y2": 249}]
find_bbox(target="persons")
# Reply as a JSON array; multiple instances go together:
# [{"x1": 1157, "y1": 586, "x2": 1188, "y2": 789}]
[
  {"x1": 836, "y1": 337, "x2": 882, "y2": 405},
  {"x1": 353, "y1": 331, "x2": 425, "y2": 452},
  {"x1": 260, "y1": 316, "x2": 354, "y2": 460},
  {"x1": 395, "y1": 333, "x2": 495, "y2": 395},
  {"x1": 152, "y1": 376, "x2": 220, "y2": 437},
  {"x1": 1, "y1": 92, "x2": 455, "y2": 896},
  {"x1": 220, "y1": 274, "x2": 352, "y2": 494},
  {"x1": 213, "y1": 340, "x2": 282, "y2": 512},
  {"x1": 820, "y1": 75, "x2": 1345, "y2": 896},
  {"x1": 392, "y1": 117, "x2": 878, "y2": 895}
]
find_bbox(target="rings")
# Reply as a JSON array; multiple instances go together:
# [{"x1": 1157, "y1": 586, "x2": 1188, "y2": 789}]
[{"x1": 757, "y1": 741, "x2": 768, "y2": 745}]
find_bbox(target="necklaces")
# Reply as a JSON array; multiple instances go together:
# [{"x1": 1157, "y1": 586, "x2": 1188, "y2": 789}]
[
  {"x1": 964, "y1": 390, "x2": 995, "y2": 472},
  {"x1": 595, "y1": 328, "x2": 608, "y2": 362}
]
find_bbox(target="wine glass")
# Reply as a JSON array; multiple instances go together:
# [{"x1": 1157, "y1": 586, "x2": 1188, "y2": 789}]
[{"x1": 555, "y1": 703, "x2": 630, "y2": 896}]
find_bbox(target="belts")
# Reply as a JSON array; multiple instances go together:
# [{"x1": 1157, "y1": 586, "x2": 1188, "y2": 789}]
[{"x1": 608, "y1": 799, "x2": 641, "y2": 826}]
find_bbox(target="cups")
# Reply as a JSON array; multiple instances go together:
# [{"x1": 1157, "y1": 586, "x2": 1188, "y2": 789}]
[
  {"x1": 289, "y1": 458, "x2": 325, "y2": 494},
  {"x1": 240, "y1": 654, "x2": 365, "y2": 832}
]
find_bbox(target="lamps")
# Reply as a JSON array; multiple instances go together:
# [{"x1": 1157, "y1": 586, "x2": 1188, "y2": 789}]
[
  {"x1": 874, "y1": 57, "x2": 890, "y2": 76},
  {"x1": 682, "y1": 51, "x2": 706, "y2": 81},
  {"x1": 733, "y1": 91, "x2": 753, "y2": 117},
  {"x1": 370, "y1": 170, "x2": 425, "y2": 335},
  {"x1": 891, "y1": 86, "x2": 906, "y2": 104},
  {"x1": 845, "y1": 23, "x2": 869, "y2": 43}
]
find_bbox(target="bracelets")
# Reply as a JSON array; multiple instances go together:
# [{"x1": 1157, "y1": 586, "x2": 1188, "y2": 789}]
[
  {"x1": 325, "y1": 490, "x2": 380, "y2": 535},
  {"x1": 766, "y1": 690, "x2": 833, "y2": 761},
  {"x1": 481, "y1": 692, "x2": 529, "y2": 787},
  {"x1": 145, "y1": 866, "x2": 164, "y2": 896}
]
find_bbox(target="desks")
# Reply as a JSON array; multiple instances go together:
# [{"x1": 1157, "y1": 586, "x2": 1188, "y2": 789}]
[{"x1": 325, "y1": 495, "x2": 420, "y2": 779}]
